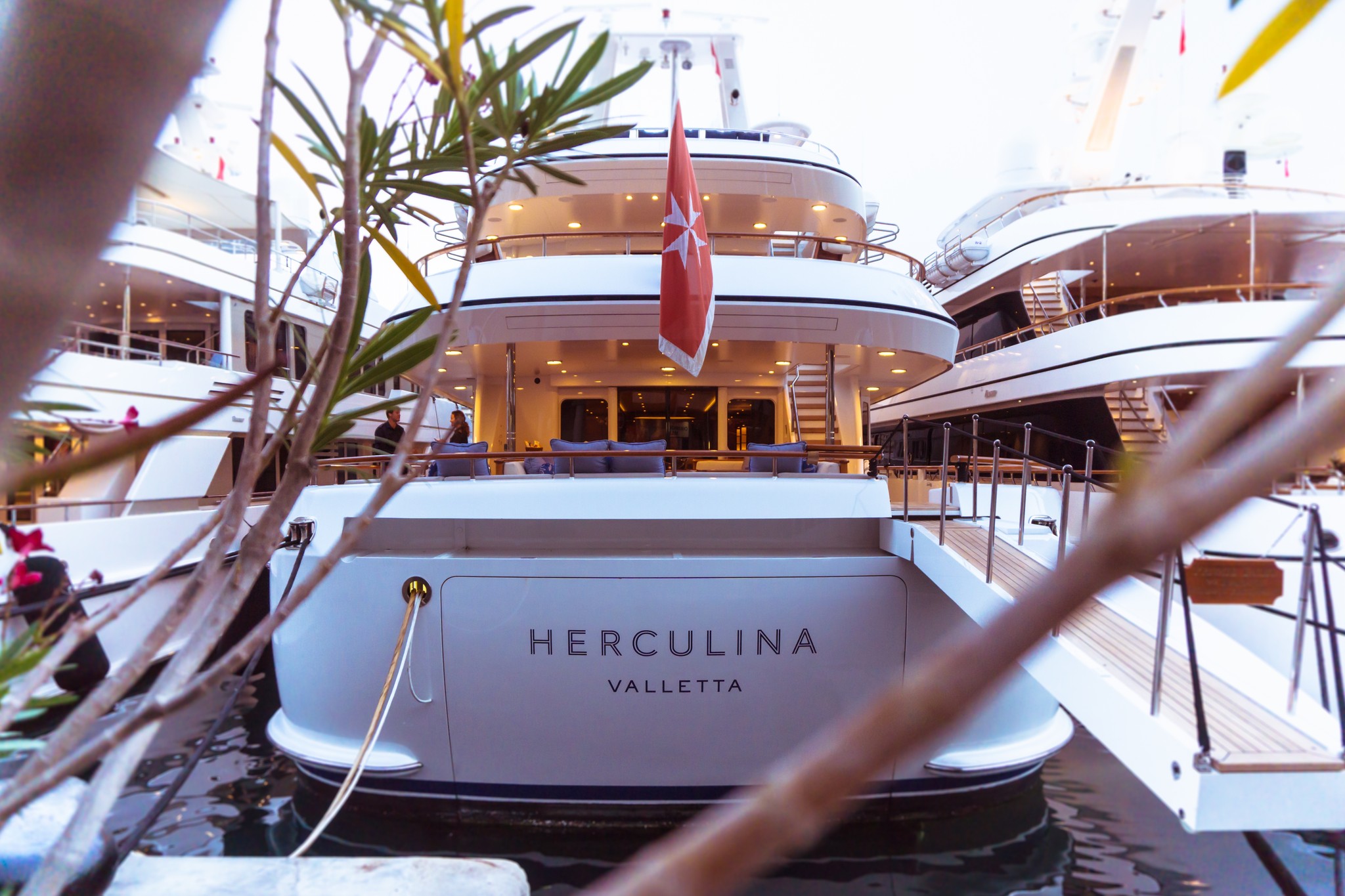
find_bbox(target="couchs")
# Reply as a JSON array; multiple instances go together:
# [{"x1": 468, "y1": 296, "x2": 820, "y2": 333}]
[{"x1": 344, "y1": 459, "x2": 850, "y2": 484}]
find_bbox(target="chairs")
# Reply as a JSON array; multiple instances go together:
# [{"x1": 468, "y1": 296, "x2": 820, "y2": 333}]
[
  {"x1": 472, "y1": 241, "x2": 504, "y2": 262},
  {"x1": 810, "y1": 237, "x2": 853, "y2": 261}
]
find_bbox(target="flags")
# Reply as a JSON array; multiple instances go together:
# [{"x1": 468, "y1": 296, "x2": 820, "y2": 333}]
[{"x1": 658, "y1": 89, "x2": 715, "y2": 377}]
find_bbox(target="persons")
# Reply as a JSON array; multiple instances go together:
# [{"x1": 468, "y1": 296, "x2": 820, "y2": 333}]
[
  {"x1": 375, "y1": 405, "x2": 408, "y2": 477},
  {"x1": 435, "y1": 410, "x2": 470, "y2": 444}
]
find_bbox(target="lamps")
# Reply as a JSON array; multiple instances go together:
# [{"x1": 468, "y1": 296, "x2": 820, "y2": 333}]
[
  {"x1": 731, "y1": 89, "x2": 740, "y2": 105},
  {"x1": 660, "y1": 54, "x2": 670, "y2": 70},
  {"x1": 681, "y1": 52, "x2": 694, "y2": 71}
]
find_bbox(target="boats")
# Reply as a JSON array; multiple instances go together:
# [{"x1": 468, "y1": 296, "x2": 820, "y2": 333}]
[
  {"x1": 262, "y1": 32, "x2": 1075, "y2": 869},
  {"x1": 0, "y1": 54, "x2": 471, "y2": 738}
]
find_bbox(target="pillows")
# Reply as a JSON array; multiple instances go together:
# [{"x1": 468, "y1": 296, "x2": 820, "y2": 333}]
[
  {"x1": 607, "y1": 440, "x2": 666, "y2": 475},
  {"x1": 741, "y1": 456, "x2": 750, "y2": 469},
  {"x1": 524, "y1": 456, "x2": 545, "y2": 475},
  {"x1": 748, "y1": 440, "x2": 807, "y2": 474},
  {"x1": 801, "y1": 457, "x2": 815, "y2": 472},
  {"x1": 431, "y1": 440, "x2": 490, "y2": 476},
  {"x1": 539, "y1": 463, "x2": 554, "y2": 475},
  {"x1": 550, "y1": 439, "x2": 610, "y2": 473}
]
